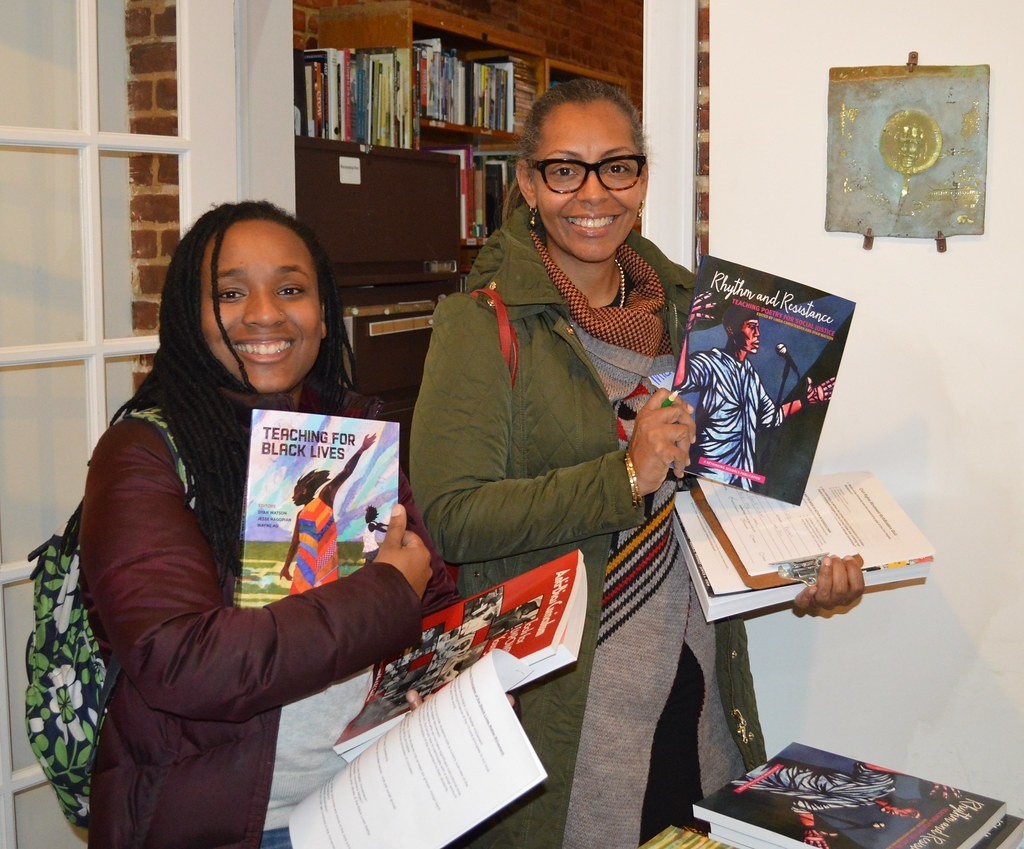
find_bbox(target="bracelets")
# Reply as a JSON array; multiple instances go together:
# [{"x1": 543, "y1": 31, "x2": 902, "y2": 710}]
[{"x1": 623, "y1": 452, "x2": 644, "y2": 510}]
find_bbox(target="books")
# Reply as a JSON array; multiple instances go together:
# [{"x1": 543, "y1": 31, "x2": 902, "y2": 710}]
[
  {"x1": 413, "y1": 34, "x2": 537, "y2": 133},
  {"x1": 289, "y1": 41, "x2": 412, "y2": 149},
  {"x1": 671, "y1": 470, "x2": 935, "y2": 621},
  {"x1": 669, "y1": 254, "x2": 855, "y2": 506},
  {"x1": 331, "y1": 551, "x2": 588, "y2": 764},
  {"x1": 637, "y1": 741, "x2": 1024, "y2": 849},
  {"x1": 421, "y1": 142, "x2": 528, "y2": 246},
  {"x1": 233, "y1": 410, "x2": 400, "y2": 610}
]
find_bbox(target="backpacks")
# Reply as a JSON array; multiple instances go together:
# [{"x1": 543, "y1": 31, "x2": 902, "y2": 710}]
[{"x1": 22, "y1": 404, "x2": 196, "y2": 827}]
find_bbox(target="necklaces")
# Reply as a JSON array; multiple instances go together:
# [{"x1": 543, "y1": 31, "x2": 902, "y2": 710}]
[{"x1": 612, "y1": 258, "x2": 626, "y2": 308}]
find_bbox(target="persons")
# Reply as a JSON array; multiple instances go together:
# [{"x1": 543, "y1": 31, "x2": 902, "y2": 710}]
[
  {"x1": 80, "y1": 201, "x2": 515, "y2": 849},
  {"x1": 407, "y1": 79, "x2": 866, "y2": 849}
]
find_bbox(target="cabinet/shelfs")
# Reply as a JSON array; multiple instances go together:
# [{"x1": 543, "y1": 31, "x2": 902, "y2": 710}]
[
  {"x1": 293, "y1": 133, "x2": 463, "y2": 491},
  {"x1": 315, "y1": 0, "x2": 633, "y2": 288}
]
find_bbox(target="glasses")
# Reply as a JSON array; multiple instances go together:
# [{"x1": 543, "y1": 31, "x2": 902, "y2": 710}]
[{"x1": 525, "y1": 151, "x2": 646, "y2": 195}]
